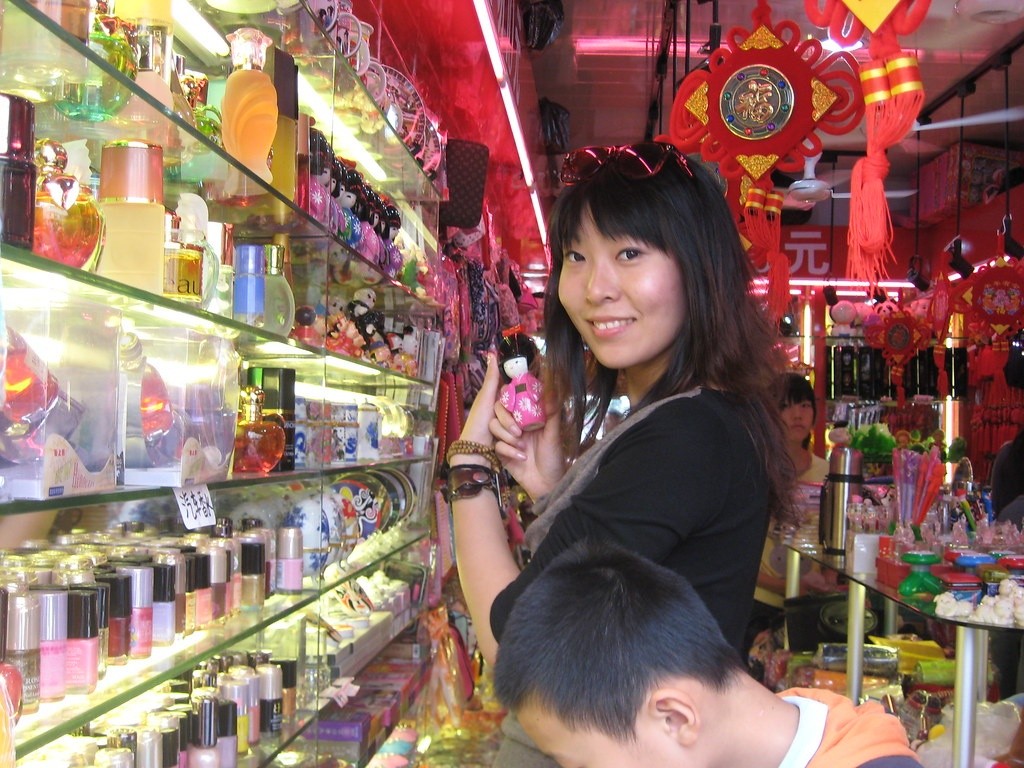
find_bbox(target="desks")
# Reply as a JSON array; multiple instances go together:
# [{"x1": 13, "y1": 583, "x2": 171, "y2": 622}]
[{"x1": 767, "y1": 525, "x2": 1024, "y2": 768}]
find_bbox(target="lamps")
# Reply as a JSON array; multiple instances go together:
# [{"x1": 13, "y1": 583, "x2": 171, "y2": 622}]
[
  {"x1": 775, "y1": 314, "x2": 792, "y2": 334},
  {"x1": 997, "y1": 57, "x2": 1024, "y2": 261},
  {"x1": 909, "y1": 126, "x2": 928, "y2": 290},
  {"x1": 824, "y1": 150, "x2": 839, "y2": 306},
  {"x1": 866, "y1": 276, "x2": 887, "y2": 302},
  {"x1": 948, "y1": 81, "x2": 975, "y2": 278}
]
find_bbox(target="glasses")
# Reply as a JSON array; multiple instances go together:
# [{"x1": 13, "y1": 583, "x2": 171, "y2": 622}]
[{"x1": 558, "y1": 141, "x2": 693, "y2": 186}]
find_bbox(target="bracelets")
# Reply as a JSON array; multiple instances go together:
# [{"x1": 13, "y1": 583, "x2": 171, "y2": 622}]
[
  {"x1": 446, "y1": 440, "x2": 500, "y2": 470},
  {"x1": 446, "y1": 464, "x2": 497, "y2": 501}
]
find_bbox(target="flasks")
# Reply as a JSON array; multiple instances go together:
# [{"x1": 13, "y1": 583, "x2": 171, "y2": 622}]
[{"x1": 817, "y1": 444, "x2": 864, "y2": 556}]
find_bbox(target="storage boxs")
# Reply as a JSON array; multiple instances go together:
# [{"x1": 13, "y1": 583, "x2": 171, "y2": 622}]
[
  {"x1": 118, "y1": 304, "x2": 245, "y2": 487},
  {"x1": 302, "y1": 630, "x2": 437, "y2": 768},
  {"x1": 1, "y1": 290, "x2": 123, "y2": 500}
]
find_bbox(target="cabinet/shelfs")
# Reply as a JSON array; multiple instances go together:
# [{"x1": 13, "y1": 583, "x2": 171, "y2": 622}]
[{"x1": 1, "y1": 14, "x2": 451, "y2": 767}]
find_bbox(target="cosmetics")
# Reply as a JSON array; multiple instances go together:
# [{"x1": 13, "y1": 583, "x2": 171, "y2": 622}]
[{"x1": 0, "y1": 2, "x2": 304, "y2": 768}]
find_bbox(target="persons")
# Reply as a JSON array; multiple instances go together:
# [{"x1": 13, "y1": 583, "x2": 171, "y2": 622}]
[
  {"x1": 987, "y1": 432, "x2": 1024, "y2": 698},
  {"x1": 449, "y1": 138, "x2": 801, "y2": 768},
  {"x1": 741, "y1": 372, "x2": 830, "y2": 694},
  {"x1": 492, "y1": 541, "x2": 923, "y2": 768}
]
